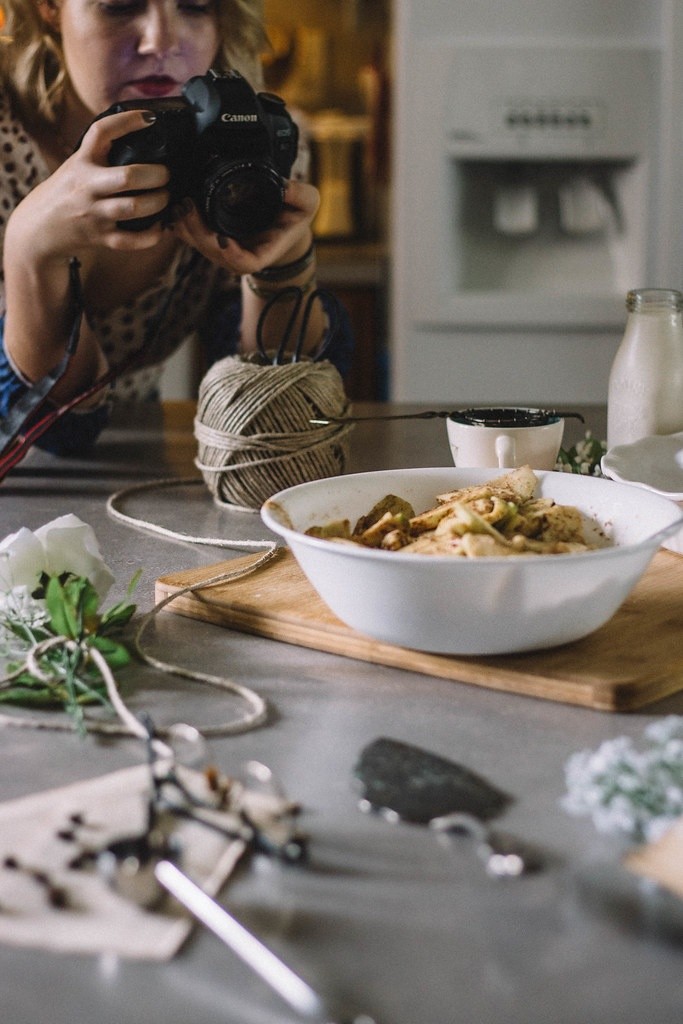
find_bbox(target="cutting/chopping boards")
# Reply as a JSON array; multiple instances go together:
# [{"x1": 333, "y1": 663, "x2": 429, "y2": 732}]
[{"x1": 158, "y1": 532, "x2": 683, "y2": 715}]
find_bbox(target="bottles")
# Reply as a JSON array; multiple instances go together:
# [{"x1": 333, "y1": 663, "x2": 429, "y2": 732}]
[{"x1": 607, "y1": 287, "x2": 683, "y2": 453}]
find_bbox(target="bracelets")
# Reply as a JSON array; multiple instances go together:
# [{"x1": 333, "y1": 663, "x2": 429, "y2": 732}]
[
  {"x1": 244, "y1": 261, "x2": 320, "y2": 301},
  {"x1": 247, "y1": 225, "x2": 318, "y2": 282}
]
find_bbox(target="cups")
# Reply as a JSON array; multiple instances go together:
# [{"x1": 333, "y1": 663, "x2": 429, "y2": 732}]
[{"x1": 445, "y1": 404, "x2": 565, "y2": 472}]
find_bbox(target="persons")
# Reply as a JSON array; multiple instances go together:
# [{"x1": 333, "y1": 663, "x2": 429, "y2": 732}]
[{"x1": 0, "y1": 0, "x2": 374, "y2": 461}]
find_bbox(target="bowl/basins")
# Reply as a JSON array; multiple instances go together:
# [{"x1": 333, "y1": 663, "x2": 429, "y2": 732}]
[
  {"x1": 601, "y1": 428, "x2": 683, "y2": 558},
  {"x1": 261, "y1": 461, "x2": 683, "y2": 658}
]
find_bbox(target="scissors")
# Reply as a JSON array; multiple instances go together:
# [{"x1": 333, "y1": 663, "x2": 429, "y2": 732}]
[{"x1": 252, "y1": 284, "x2": 349, "y2": 368}]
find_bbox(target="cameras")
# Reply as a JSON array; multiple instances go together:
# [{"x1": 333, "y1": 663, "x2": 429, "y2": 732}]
[{"x1": 76, "y1": 66, "x2": 300, "y2": 249}]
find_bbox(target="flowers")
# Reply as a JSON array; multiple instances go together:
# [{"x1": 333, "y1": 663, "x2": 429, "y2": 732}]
[{"x1": 1, "y1": 512, "x2": 153, "y2": 738}]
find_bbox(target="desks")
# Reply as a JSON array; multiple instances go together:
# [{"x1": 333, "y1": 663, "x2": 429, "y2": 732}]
[{"x1": 1, "y1": 397, "x2": 683, "y2": 1022}]
